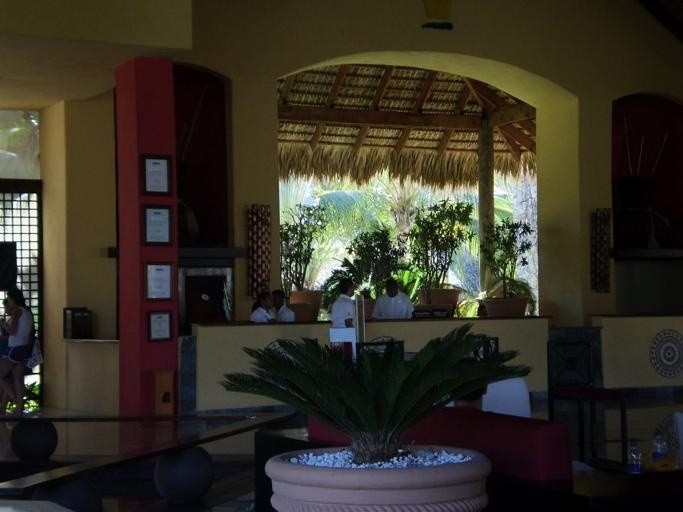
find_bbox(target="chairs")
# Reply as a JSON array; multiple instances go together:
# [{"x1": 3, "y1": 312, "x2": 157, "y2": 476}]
[{"x1": 547, "y1": 324, "x2": 628, "y2": 470}]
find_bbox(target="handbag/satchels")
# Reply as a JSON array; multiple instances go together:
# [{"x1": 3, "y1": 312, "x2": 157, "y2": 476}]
[{"x1": 27, "y1": 339, "x2": 44, "y2": 368}]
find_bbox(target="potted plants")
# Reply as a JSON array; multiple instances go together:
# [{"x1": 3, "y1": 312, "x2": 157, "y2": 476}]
[
  {"x1": 280, "y1": 195, "x2": 534, "y2": 320},
  {"x1": 216, "y1": 320, "x2": 533, "y2": 512}
]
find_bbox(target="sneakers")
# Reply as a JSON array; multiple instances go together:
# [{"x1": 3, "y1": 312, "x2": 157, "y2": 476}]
[{"x1": 10, "y1": 408, "x2": 23, "y2": 416}]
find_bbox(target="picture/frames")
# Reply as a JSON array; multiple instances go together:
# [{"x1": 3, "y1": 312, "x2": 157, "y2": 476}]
[
  {"x1": 146, "y1": 310, "x2": 172, "y2": 342},
  {"x1": 143, "y1": 154, "x2": 171, "y2": 195},
  {"x1": 144, "y1": 261, "x2": 173, "y2": 302},
  {"x1": 142, "y1": 205, "x2": 172, "y2": 246}
]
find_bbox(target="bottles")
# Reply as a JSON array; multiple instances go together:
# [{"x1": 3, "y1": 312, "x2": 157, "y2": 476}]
[
  {"x1": 650, "y1": 429, "x2": 668, "y2": 462},
  {"x1": 627, "y1": 440, "x2": 642, "y2": 473}
]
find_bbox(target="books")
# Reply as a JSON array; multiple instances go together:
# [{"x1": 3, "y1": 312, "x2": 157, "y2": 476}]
[{"x1": 63, "y1": 307, "x2": 91, "y2": 339}]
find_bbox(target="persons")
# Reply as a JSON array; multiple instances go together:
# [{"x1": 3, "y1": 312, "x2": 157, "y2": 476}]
[
  {"x1": 0, "y1": 288, "x2": 35, "y2": 417},
  {"x1": 271, "y1": 290, "x2": 295, "y2": 322},
  {"x1": 249, "y1": 292, "x2": 277, "y2": 323},
  {"x1": 371, "y1": 277, "x2": 416, "y2": 322},
  {"x1": 331, "y1": 278, "x2": 356, "y2": 328}
]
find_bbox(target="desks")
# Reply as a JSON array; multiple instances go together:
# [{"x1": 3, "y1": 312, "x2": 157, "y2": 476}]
[{"x1": 2, "y1": 410, "x2": 297, "y2": 512}]
